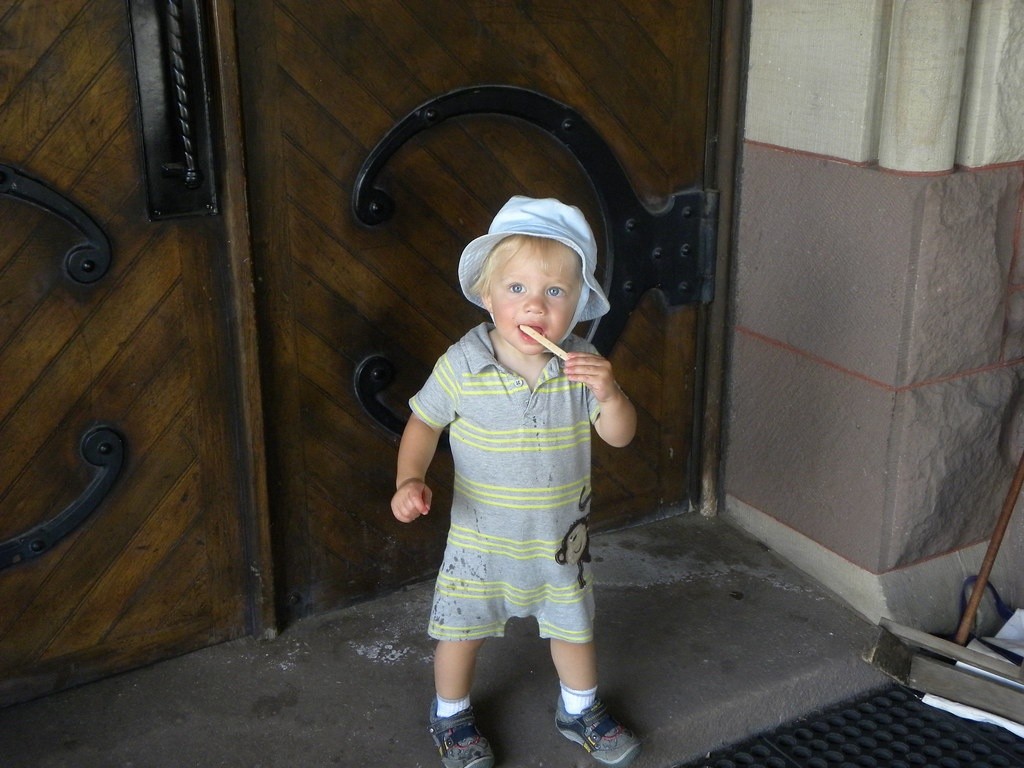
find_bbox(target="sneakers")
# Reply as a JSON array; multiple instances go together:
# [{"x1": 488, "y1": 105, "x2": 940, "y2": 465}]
[
  {"x1": 426, "y1": 698, "x2": 495, "y2": 768},
  {"x1": 555, "y1": 694, "x2": 642, "y2": 767}
]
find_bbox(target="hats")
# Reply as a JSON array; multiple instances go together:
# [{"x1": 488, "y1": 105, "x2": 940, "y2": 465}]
[{"x1": 457, "y1": 195, "x2": 611, "y2": 321}]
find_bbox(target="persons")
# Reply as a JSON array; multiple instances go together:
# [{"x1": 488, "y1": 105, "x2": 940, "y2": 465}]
[{"x1": 392, "y1": 190, "x2": 641, "y2": 768}]
